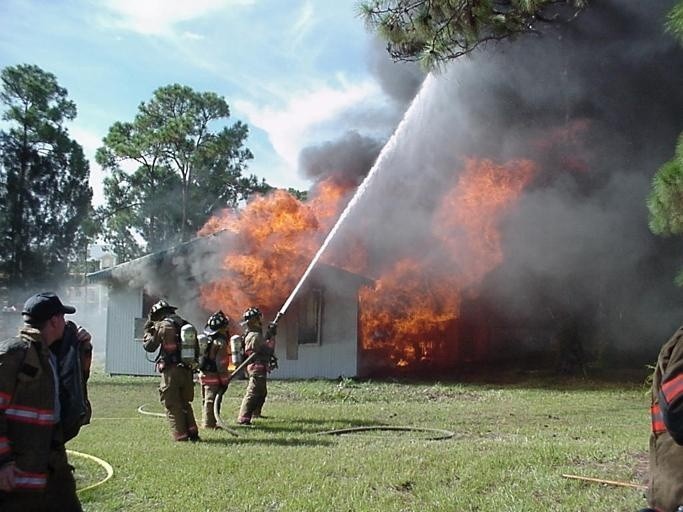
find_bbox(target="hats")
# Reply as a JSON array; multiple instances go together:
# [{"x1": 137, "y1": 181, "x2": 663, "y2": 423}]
[{"x1": 21, "y1": 291, "x2": 76, "y2": 324}]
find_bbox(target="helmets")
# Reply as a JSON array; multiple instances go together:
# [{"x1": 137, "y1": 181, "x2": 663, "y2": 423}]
[
  {"x1": 207, "y1": 310, "x2": 229, "y2": 331},
  {"x1": 243, "y1": 307, "x2": 260, "y2": 321},
  {"x1": 150, "y1": 299, "x2": 178, "y2": 321}
]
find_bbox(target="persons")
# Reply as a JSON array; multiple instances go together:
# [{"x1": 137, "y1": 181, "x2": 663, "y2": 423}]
[
  {"x1": 0, "y1": 290, "x2": 95, "y2": 511},
  {"x1": 141, "y1": 298, "x2": 205, "y2": 442},
  {"x1": 237, "y1": 306, "x2": 275, "y2": 425},
  {"x1": 194, "y1": 309, "x2": 233, "y2": 430}
]
